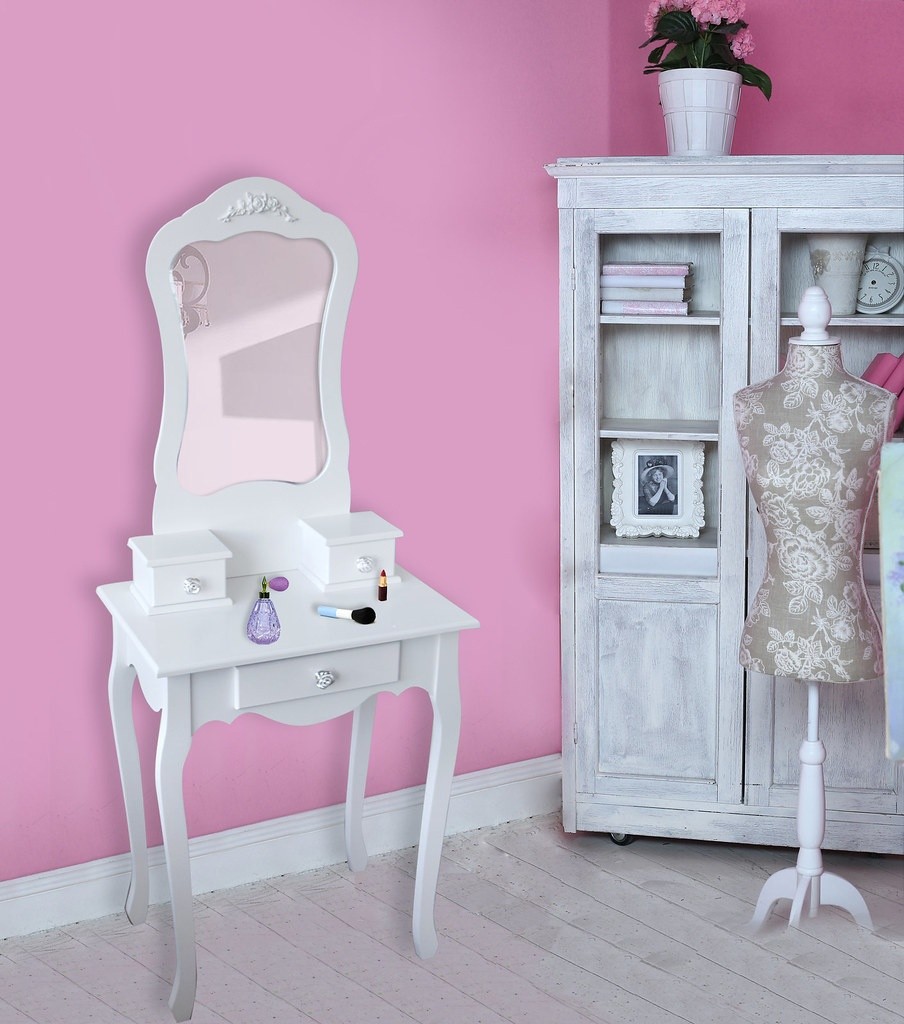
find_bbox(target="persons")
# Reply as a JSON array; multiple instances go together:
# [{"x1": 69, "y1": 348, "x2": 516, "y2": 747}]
[
  {"x1": 732, "y1": 334, "x2": 895, "y2": 684},
  {"x1": 640, "y1": 459, "x2": 676, "y2": 506}
]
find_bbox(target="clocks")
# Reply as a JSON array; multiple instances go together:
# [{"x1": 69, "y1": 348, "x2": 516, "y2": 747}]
[{"x1": 855, "y1": 244, "x2": 904, "y2": 314}]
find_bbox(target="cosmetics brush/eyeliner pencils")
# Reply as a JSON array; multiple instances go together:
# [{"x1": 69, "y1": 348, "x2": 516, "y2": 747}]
[{"x1": 318, "y1": 604, "x2": 375, "y2": 625}]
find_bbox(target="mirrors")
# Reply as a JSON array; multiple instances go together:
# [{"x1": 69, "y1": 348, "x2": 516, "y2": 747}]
[{"x1": 146, "y1": 178, "x2": 359, "y2": 576}]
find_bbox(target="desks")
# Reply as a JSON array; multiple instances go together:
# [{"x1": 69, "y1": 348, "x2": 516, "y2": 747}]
[{"x1": 95, "y1": 511, "x2": 480, "y2": 1023}]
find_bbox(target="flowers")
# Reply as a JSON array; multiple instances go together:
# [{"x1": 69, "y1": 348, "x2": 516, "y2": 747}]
[{"x1": 638, "y1": 0, "x2": 772, "y2": 105}]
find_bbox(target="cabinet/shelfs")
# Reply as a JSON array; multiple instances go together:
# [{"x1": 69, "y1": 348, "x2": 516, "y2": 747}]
[{"x1": 543, "y1": 155, "x2": 904, "y2": 855}]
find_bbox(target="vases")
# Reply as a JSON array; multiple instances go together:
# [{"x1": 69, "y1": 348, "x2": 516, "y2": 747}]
[{"x1": 658, "y1": 68, "x2": 743, "y2": 156}]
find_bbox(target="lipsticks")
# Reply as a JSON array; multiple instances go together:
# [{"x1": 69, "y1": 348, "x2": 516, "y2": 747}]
[{"x1": 378, "y1": 570, "x2": 388, "y2": 601}]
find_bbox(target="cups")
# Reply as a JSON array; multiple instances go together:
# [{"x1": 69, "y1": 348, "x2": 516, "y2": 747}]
[{"x1": 808, "y1": 233, "x2": 869, "y2": 317}]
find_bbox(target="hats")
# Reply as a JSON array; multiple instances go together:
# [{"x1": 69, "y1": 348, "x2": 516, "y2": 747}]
[{"x1": 641, "y1": 457, "x2": 674, "y2": 482}]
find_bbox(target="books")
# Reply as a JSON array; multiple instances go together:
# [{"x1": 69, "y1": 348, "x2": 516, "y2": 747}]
[
  {"x1": 600, "y1": 261, "x2": 694, "y2": 317},
  {"x1": 861, "y1": 351, "x2": 904, "y2": 441}
]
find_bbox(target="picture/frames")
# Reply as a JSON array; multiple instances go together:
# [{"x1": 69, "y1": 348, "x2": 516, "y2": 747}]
[{"x1": 609, "y1": 439, "x2": 705, "y2": 539}]
